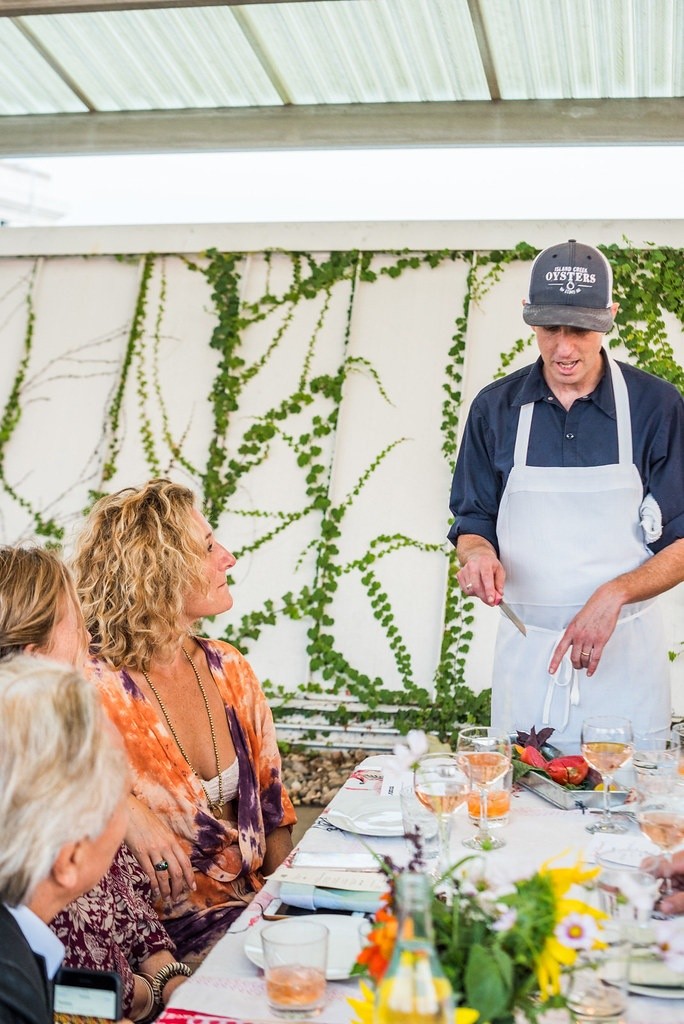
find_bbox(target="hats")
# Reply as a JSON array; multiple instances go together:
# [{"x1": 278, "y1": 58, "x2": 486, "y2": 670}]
[{"x1": 522, "y1": 238, "x2": 614, "y2": 332}]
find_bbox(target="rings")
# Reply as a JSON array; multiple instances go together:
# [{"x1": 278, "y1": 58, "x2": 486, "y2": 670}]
[
  {"x1": 153, "y1": 860, "x2": 167, "y2": 871},
  {"x1": 466, "y1": 584, "x2": 471, "y2": 588},
  {"x1": 581, "y1": 652, "x2": 590, "y2": 656}
]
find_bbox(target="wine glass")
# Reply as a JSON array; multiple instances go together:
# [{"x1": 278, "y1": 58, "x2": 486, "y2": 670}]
[
  {"x1": 581, "y1": 716, "x2": 634, "y2": 839},
  {"x1": 414, "y1": 753, "x2": 472, "y2": 883},
  {"x1": 457, "y1": 727, "x2": 511, "y2": 851}
]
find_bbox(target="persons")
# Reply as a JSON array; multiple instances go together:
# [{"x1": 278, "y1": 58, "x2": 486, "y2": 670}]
[
  {"x1": 1, "y1": 479, "x2": 299, "y2": 1023},
  {"x1": 641, "y1": 851, "x2": 684, "y2": 914},
  {"x1": 448, "y1": 239, "x2": 684, "y2": 745}
]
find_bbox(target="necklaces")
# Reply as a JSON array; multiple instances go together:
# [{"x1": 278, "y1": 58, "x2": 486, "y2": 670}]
[{"x1": 142, "y1": 645, "x2": 224, "y2": 820}]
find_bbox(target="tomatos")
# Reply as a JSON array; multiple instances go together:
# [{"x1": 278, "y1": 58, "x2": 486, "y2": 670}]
[{"x1": 522, "y1": 745, "x2": 589, "y2": 783}]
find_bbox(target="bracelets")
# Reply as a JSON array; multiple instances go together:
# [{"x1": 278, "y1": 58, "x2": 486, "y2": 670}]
[
  {"x1": 131, "y1": 972, "x2": 158, "y2": 1023},
  {"x1": 153, "y1": 962, "x2": 193, "y2": 1010}
]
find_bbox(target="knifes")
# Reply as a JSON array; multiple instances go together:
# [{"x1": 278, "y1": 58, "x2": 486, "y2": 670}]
[{"x1": 494, "y1": 590, "x2": 526, "y2": 637}]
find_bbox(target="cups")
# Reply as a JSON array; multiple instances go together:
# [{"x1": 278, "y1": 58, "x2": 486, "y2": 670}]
[
  {"x1": 638, "y1": 781, "x2": 684, "y2": 920},
  {"x1": 673, "y1": 723, "x2": 684, "y2": 786},
  {"x1": 400, "y1": 785, "x2": 449, "y2": 861},
  {"x1": 596, "y1": 840, "x2": 662, "y2": 932},
  {"x1": 563, "y1": 917, "x2": 629, "y2": 1021},
  {"x1": 261, "y1": 920, "x2": 329, "y2": 1018},
  {"x1": 633, "y1": 739, "x2": 680, "y2": 802},
  {"x1": 467, "y1": 764, "x2": 513, "y2": 828}
]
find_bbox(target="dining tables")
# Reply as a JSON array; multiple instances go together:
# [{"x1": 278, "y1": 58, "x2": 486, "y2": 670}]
[{"x1": 156, "y1": 754, "x2": 684, "y2": 1024}]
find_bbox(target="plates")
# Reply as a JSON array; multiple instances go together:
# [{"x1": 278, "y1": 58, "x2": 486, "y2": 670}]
[
  {"x1": 601, "y1": 973, "x2": 684, "y2": 999},
  {"x1": 327, "y1": 797, "x2": 405, "y2": 836},
  {"x1": 244, "y1": 914, "x2": 374, "y2": 980},
  {"x1": 600, "y1": 923, "x2": 684, "y2": 998}
]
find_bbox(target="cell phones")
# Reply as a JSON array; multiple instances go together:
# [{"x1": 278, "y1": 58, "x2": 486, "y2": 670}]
[
  {"x1": 51, "y1": 967, "x2": 125, "y2": 1024},
  {"x1": 262, "y1": 898, "x2": 366, "y2": 922}
]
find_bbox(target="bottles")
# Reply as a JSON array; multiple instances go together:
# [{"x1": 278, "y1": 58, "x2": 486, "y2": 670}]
[{"x1": 375, "y1": 873, "x2": 455, "y2": 1024}]
